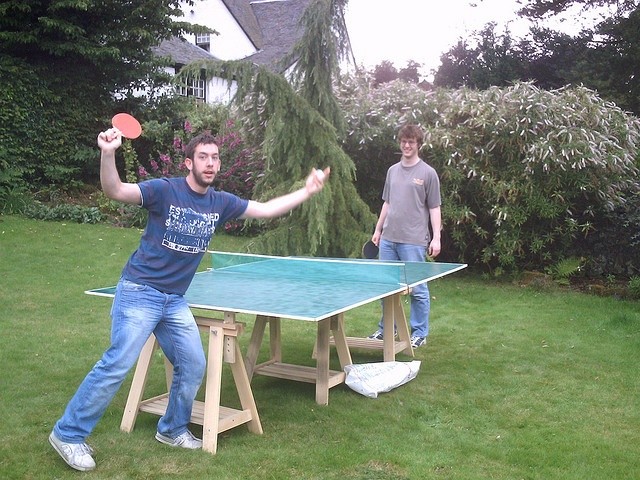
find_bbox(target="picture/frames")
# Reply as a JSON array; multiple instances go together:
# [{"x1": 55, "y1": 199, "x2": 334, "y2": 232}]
[{"x1": 84, "y1": 255, "x2": 468, "y2": 450}]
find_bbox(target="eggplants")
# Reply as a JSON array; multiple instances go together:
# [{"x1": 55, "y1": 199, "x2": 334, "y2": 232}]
[
  {"x1": 112, "y1": 113, "x2": 142, "y2": 139},
  {"x1": 362, "y1": 240, "x2": 379, "y2": 259}
]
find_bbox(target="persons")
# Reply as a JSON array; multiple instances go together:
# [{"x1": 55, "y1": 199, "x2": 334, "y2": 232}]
[
  {"x1": 47, "y1": 125, "x2": 332, "y2": 472},
  {"x1": 366, "y1": 124, "x2": 442, "y2": 348}
]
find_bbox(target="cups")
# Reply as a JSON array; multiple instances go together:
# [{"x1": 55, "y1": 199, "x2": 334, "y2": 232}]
[{"x1": 401, "y1": 139, "x2": 418, "y2": 145}]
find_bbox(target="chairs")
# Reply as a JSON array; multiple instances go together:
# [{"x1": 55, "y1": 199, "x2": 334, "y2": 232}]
[
  {"x1": 154, "y1": 428, "x2": 204, "y2": 450},
  {"x1": 366, "y1": 329, "x2": 398, "y2": 341},
  {"x1": 48, "y1": 429, "x2": 97, "y2": 472},
  {"x1": 410, "y1": 336, "x2": 426, "y2": 348}
]
